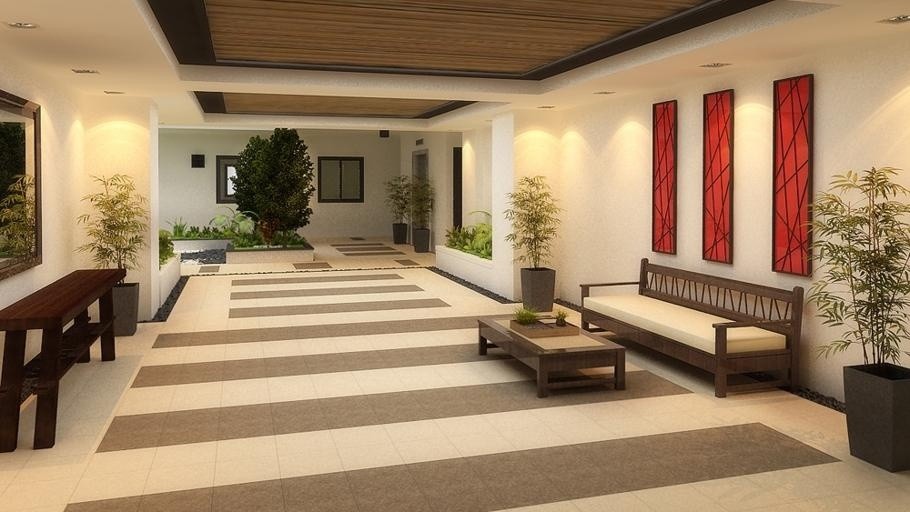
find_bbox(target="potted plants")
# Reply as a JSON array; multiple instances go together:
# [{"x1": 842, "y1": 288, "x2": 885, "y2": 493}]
[
  {"x1": 384, "y1": 175, "x2": 410, "y2": 244},
  {"x1": 802, "y1": 162, "x2": 909, "y2": 475},
  {"x1": 503, "y1": 175, "x2": 567, "y2": 314},
  {"x1": 77, "y1": 172, "x2": 143, "y2": 336},
  {"x1": 404, "y1": 179, "x2": 432, "y2": 254}
]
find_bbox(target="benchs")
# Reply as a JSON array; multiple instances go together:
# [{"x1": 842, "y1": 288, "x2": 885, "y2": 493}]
[{"x1": 579, "y1": 256, "x2": 804, "y2": 399}]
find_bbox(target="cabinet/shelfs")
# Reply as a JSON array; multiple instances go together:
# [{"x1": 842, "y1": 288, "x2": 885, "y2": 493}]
[{"x1": 0, "y1": 270, "x2": 130, "y2": 452}]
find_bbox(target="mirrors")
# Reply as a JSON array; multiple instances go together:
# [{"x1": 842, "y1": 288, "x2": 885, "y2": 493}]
[{"x1": 1, "y1": 89, "x2": 43, "y2": 280}]
